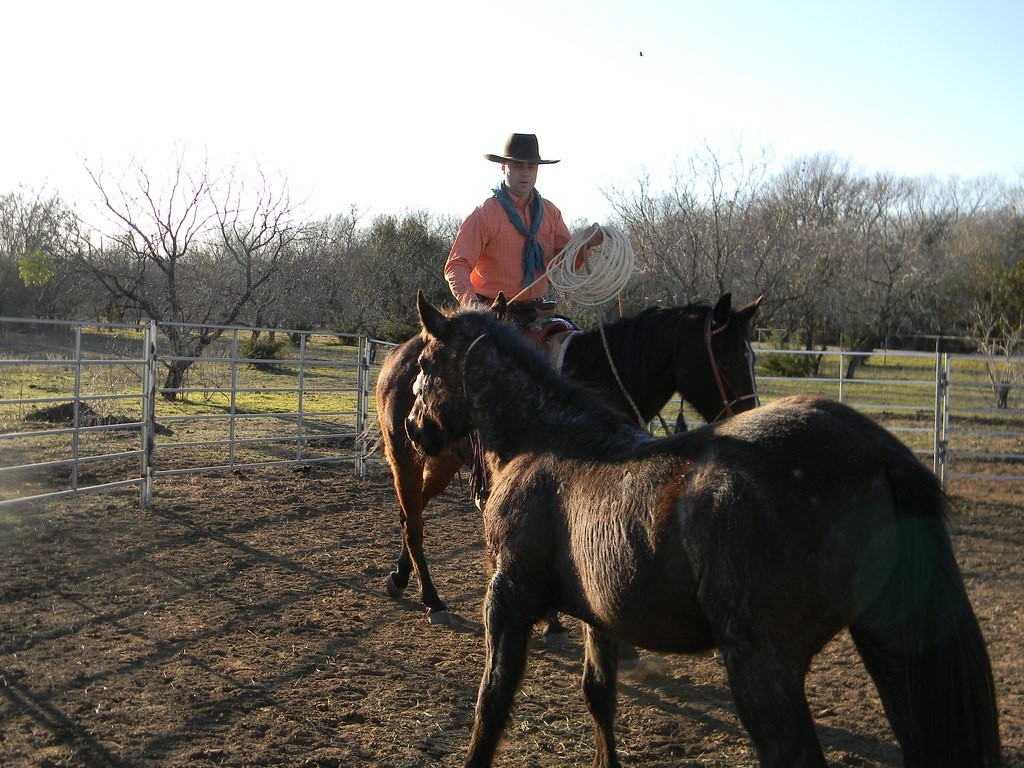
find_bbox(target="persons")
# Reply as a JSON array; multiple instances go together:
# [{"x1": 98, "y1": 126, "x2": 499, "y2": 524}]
[{"x1": 444, "y1": 133, "x2": 603, "y2": 510}]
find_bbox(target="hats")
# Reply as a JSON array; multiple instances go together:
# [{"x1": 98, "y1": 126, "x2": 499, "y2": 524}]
[{"x1": 484, "y1": 133, "x2": 560, "y2": 164}]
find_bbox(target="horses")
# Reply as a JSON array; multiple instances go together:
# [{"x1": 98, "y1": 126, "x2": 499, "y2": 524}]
[{"x1": 352, "y1": 288, "x2": 1005, "y2": 767}]
